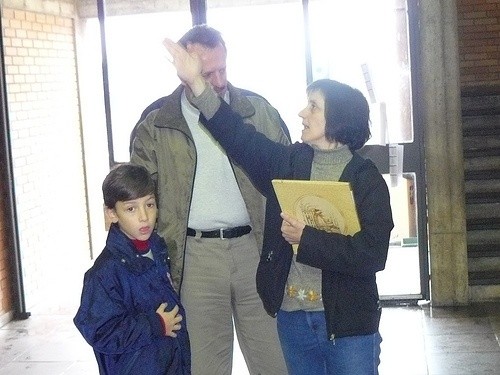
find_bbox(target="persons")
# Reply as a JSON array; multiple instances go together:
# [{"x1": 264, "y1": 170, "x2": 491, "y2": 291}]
[
  {"x1": 164, "y1": 39, "x2": 395, "y2": 375},
  {"x1": 129, "y1": 25, "x2": 292, "y2": 375},
  {"x1": 73, "y1": 165, "x2": 191, "y2": 374}
]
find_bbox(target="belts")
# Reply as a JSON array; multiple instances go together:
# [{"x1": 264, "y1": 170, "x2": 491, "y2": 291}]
[{"x1": 186, "y1": 225, "x2": 252, "y2": 238}]
